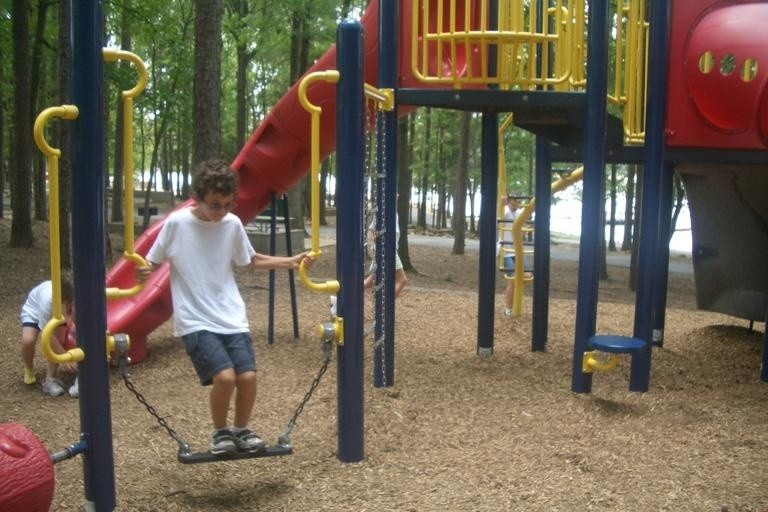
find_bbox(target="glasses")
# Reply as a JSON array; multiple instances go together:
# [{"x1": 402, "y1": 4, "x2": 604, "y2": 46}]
[{"x1": 197, "y1": 195, "x2": 238, "y2": 211}]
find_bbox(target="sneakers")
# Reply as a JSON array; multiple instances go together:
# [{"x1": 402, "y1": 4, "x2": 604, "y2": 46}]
[
  {"x1": 329, "y1": 296, "x2": 337, "y2": 321},
  {"x1": 209, "y1": 430, "x2": 240, "y2": 456},
  {"x1": 68, "y1": 376, "x2": 80, "y2": 398},
  {"x1": 41, "y1": 377, "x2": 65, "y2": 397},
  {"x1": 231, "y1": 429, "x2": 265, "y2": 455},
  {"x1": 23, "y1": 367, "x2": 36, "y2": 385}
]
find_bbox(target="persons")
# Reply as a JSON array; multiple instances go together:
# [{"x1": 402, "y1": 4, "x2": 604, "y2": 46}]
[
  {"x1": 494, "y1": 189, "x2": 535, "y2": 314},
  {"x1": 136, "y1": 158, "x2": 319, "y2": 455},
  {"x1": 329, "y1": 206, "x2": 408, "y2": 322},
  {"x1": 18, "y1": 269, "x2": 75, "y2": 395}
]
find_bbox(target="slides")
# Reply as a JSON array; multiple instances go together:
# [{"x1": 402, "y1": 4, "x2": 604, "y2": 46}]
[
  {"x1": 686, "y1": 0, "x2": 768, "y2": 145},
  {"x1": 62, "y1": 0, "x2": 489, "y2": 373}
]
